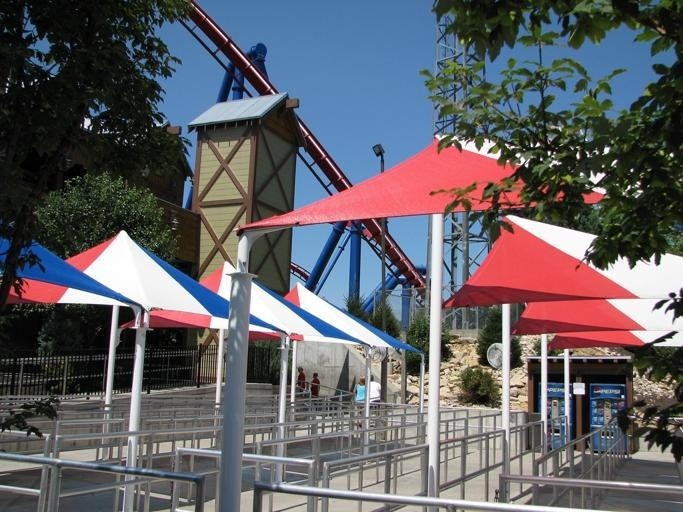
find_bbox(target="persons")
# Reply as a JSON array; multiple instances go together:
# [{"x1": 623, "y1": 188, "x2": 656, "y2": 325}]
[
  {"x1": 370, "y1": 375, "x2": 381, "y2": 427},
  {"x1": 310, "y1": 372, "x2": 320, "y2": 398},
  {"x1": 350, "y1": 375, "x2": 365, "y2": 427},
  {"x1": 296, "y1": 366, "x2": 305, "y2": 392}
]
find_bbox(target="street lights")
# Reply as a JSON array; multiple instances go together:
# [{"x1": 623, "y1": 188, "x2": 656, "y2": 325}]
[{"x1": 372, "y1": 142, "x2": 387, "y2": 333}]
